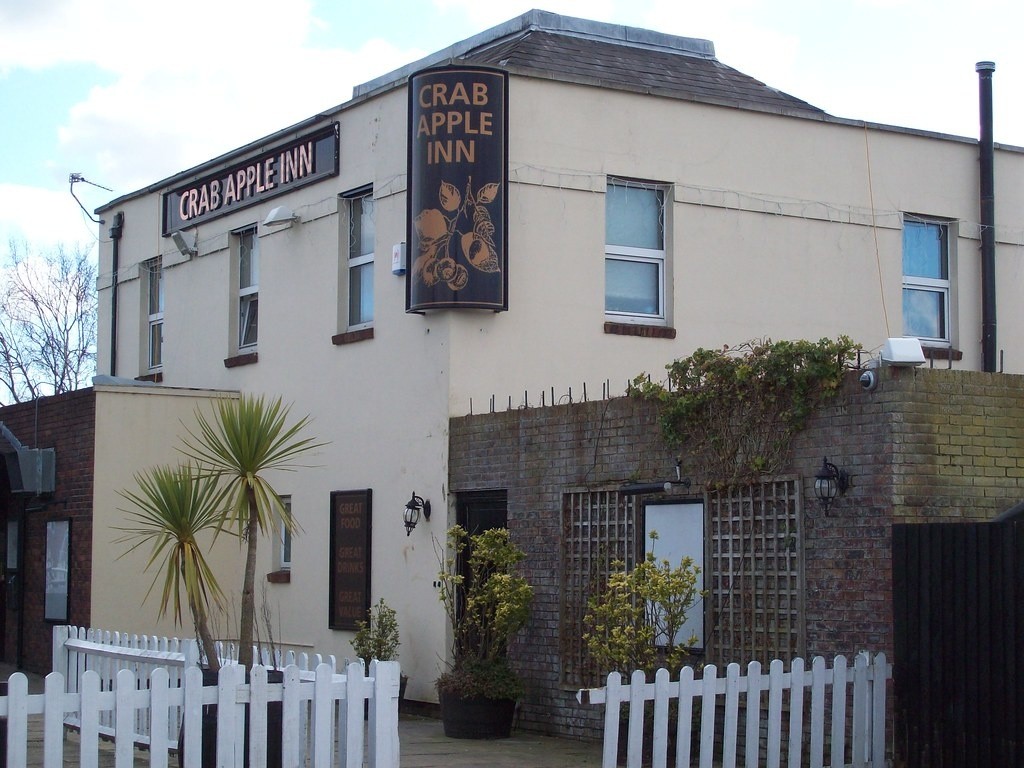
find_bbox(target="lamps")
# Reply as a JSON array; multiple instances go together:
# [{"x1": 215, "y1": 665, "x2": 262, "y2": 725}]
[
  {"x1": 813, "y1": 455, "x2": 849, "y2": 518},
  {"x1": 402, "y1": 490, "x2": 432, "y2": 539},
  {"x1": 263, "y1": 206, "x2": 302, "y2": 228},
  {"x1": 881, "y1": 334, "x2": 927, "y2": 368},
  {"x1": 172, "y1": 230, "x2": 198, "y2": 257}
]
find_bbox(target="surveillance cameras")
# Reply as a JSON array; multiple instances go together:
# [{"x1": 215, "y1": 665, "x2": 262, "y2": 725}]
[{"x1": 860, "y1": 371, "x2": 878, "y2": 390}]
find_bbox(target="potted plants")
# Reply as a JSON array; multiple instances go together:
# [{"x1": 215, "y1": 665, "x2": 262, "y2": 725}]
[
  {"x1": 349, "y1": 598, "x2": 410, "y2": 721},
  {"x1": 580, "y1": 529, "x2": 710, "y2": 767},
  {"x1": 126, "y1": 393, "x2": 334, "y2": 768},
  {"x1": 429, "y1": 525, "x2": 537, "y2": 741}
]
